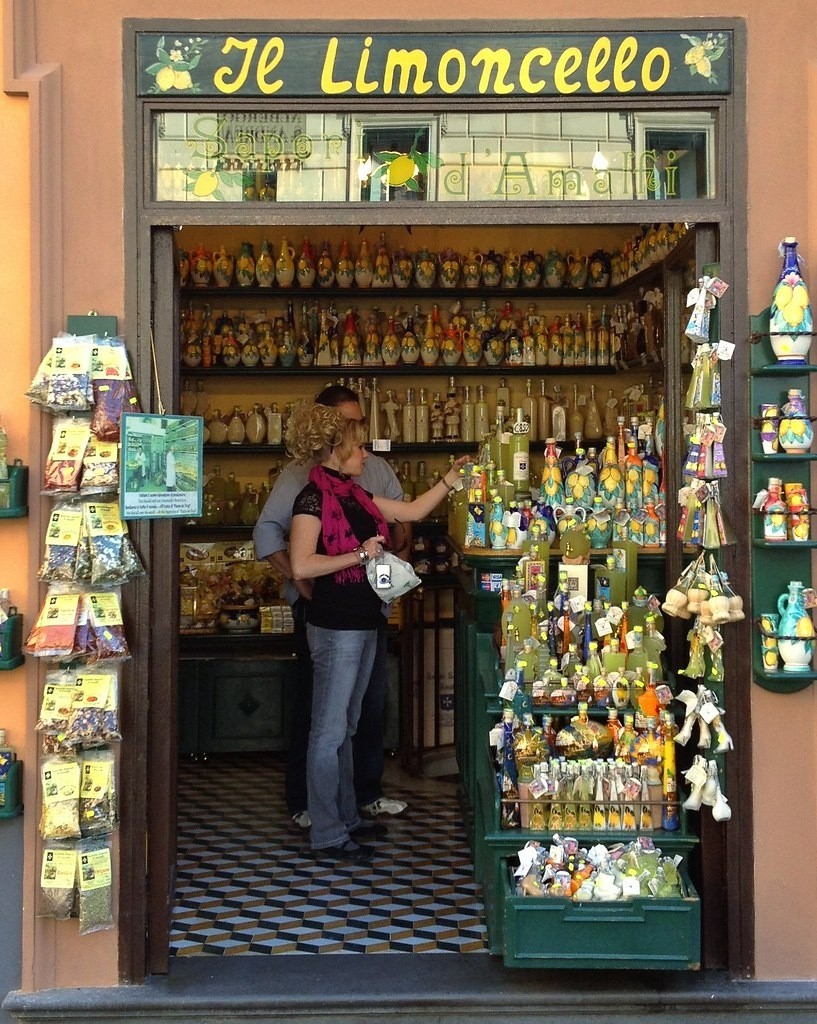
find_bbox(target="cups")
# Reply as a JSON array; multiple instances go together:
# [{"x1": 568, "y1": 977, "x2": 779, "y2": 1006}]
[
  {"x1": 760, "y1": 404, "x2": 779, "y2": 454},
  {"x1": 784, "y1": 482, "x2": 810, "y2": 542},
  {"x1": 761, "y1": 613, "x2": 779, "y2": 670}
]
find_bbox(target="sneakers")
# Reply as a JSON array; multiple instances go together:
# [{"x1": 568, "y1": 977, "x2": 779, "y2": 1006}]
[
  {"x1": 359, "y1": 797, "x2": 409, "y2": 817},
  {"x1": 291, "y1": 810, "x2": 312, "y2": 828}
]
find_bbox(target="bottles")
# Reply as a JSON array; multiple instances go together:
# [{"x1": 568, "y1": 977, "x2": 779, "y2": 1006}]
[
  {"x1": 499, "y1": 702, "x2": 678, "y2": 831},
  {"x1": 768, "y1": 237, "x2": 814, "y2": 360},
  {"x1": 515, "y1": 833, "x2": 681, "y2": 902},
  {"x1": 374, "y1": 530, "x2": 384, "y2": 583},
  {"x1": 175, "y1": 222, "x2": 704, "y2": 288},
  {"x1": 203, "y1": 459, "x2": 284, "y2": 526},
  {"x1": 389, "y1": 458, "x2": 443, "y2": 524},
  {"x1": 684, "y1": 343, "x2": 721, "y2": 410},
  {"x1": 764, "y1": 478, "x2": 787, "y2": 536},
  {"x1": 259, "y1": 174, "x2": 275, "y2": 201},
  {"x1": 682, "y1": 412, "x2": 727, "y2": 478},
  {"x1": 712, "y1": 783, "x2": 732, "y2": 822},
  {"x1": 661, "y1": 561, "x2": 745, "y2": 624},
  {"x1": 779, "y1": 388, "x2": 814, "y2": 449},
  {"x1": 676, "y1": 478, "x2": 728, "y2": 553},
  {"x1": 499, "y1": 524, "x2": 666, "y2": 686},
  {"x1": 463, "y1": 403, "x2": 667, "y2": 550},
  {"x1": 683, "y1": 275, "x2": 710, "y2": 343},
  {"x1": 177, "y1": 289, "x2": 667, "y2": 366},
  {"x1": 179, "y1": 375, "x2": 667, "y2": 444}
]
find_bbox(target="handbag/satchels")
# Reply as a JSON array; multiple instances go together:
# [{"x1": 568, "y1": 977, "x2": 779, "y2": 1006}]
[{"x1": 366, "y1": 549, "x2": 422, "y2": 604}]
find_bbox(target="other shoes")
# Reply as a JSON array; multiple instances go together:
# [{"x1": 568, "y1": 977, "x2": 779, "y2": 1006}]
[
  {"x1": 319, "y1": 841, "x2": 376, "y2": 861},
  {"x1": 349, "y1": 824, "x2": 389, "y2": 839}
]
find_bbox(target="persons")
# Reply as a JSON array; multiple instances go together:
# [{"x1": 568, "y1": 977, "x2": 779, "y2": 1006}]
[
  {"x1": 252, "y1": 385, "x2": 410, "y2": 832},
  {"x1": 284, "y1": 403, "x2": 478, "y2": 862},
  {"x1": 166, "y1": 446, "x2": 178, "y2": 492},
  {"x1": 135, "y1": 445, "x2": 146, "y2": 487}
]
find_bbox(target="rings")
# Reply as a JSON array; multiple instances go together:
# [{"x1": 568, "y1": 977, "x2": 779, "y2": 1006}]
[{"x1": 377, "y1": 548, "x2": 381, "y2": 554}]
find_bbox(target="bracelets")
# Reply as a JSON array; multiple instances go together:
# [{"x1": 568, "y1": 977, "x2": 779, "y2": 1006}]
[
  {"x1": 442, "y1": 478, "x2": 453, "y2": 491},
  {"x1": 353, "y1": 545, "x2": 370, "y2": 567}
]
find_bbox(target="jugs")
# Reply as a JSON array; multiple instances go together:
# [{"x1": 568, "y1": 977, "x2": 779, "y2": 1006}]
[{"x1": 777, "y1": 581, "x2": 816, "y2": 665}]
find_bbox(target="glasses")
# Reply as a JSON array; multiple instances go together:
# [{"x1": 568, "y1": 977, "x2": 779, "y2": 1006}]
[
  {"x1": 349, "y1": 416, "x2": 368, "y2": 424},
  {"x1": 376, "y1": 518, "x2": 408, "y2": 553}
]
